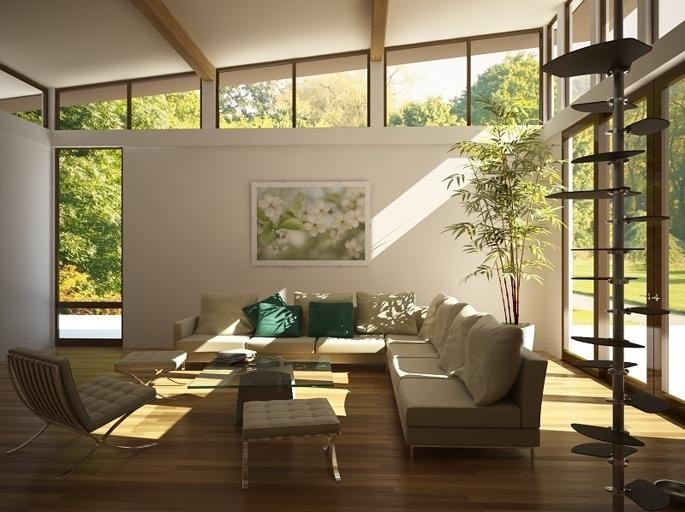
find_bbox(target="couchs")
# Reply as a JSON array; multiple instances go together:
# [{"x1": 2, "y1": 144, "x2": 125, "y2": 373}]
[{"x1": 172, "y1": 291, "x2": 548, "y2": 466}]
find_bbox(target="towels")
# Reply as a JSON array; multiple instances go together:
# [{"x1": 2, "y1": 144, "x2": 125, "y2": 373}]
[{"x1": 214, "y1": 348, "x2": 258, "y2": 368}]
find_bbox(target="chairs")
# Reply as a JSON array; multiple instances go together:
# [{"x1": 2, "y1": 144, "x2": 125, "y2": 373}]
[{"x1": 8, "y1": 349, "x2": 162, "y2": 479}]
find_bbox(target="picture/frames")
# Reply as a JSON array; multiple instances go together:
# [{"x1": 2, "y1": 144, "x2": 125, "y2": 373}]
[{"x1": 250, "y1": 181, "x2": 373, "y2": 267}]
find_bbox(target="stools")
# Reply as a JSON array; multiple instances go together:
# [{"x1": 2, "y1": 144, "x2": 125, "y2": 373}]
[
  {"x1": 240, "y1": 397, "x2": 343, "y2": 490},
  {"x1": 115, "y1": 348, "x2": 188, "y2": 400}
]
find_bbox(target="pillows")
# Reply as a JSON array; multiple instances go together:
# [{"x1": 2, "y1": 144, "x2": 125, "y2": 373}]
[
  {"x1": 192, "y1": 290, "x2": 417, "y2": 340},
  {"x1": 418, "y1": 292, "x2": 524, "y2": 408}
]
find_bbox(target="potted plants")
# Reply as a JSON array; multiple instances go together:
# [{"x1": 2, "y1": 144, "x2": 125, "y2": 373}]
[{"x1": 440, "y1": 91, "x2": 572, "y2": 351}]
